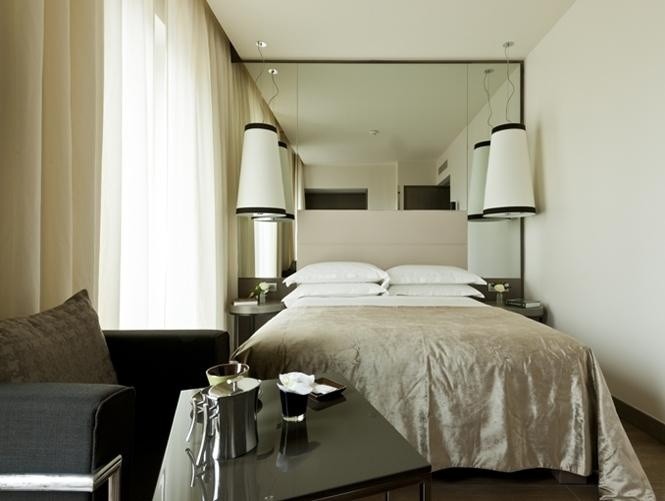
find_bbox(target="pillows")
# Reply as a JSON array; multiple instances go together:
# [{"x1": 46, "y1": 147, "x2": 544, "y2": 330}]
[
  {"x1": 282, "y1": 261, "x2": 488, "y2": 309},
  {"x1": 0, "y1": 290, "x2": 120, "y2": 385}
]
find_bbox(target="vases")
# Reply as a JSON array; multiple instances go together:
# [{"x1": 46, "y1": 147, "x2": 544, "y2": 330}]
[
  {"x1": 260, "y1": 294, "x2": 266, "y2": 305},
  {"x1": 496, "y1": 294, "x2": 503, "y2": 305}
]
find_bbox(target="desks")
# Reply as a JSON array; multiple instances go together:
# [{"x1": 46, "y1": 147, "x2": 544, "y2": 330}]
[{"x1": 152, "y1": 372, "x2": 431, "y2": 501}]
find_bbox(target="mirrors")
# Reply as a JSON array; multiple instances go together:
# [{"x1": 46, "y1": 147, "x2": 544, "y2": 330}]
[{"x1": 232, "y1": 60, "x2": 522, "y2": 279}]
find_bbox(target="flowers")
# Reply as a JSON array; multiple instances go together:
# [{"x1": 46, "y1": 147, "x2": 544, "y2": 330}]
[
  {"x1": 248, "y1": 281, "x2": 270, "y2": 299},
  {"x1": 493, "y1": 282, "x2": 510, "y2": 294}
]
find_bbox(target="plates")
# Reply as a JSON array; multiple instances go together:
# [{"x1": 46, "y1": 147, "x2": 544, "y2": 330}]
[{"x1": 310, "y1": 378, "x2": 347, "y2": 400}]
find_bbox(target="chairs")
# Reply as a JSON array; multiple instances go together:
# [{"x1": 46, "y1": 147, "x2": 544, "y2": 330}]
[{"x1": 0, "y1": 330, "x2": 229, "y2": 501}]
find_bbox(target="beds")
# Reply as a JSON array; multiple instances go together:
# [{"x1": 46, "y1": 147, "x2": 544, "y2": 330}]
[{"x1": 229, "y1": 210, "x2": 656, "y2": 501}]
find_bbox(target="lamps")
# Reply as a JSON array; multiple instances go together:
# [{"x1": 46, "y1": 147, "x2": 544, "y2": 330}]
[
  {"x1": 250, "y1": 141, "x2": 295, "y2": 221},
  {"x1": 483, "y1": 123, "x2": 536, "y2": 219},
  {"x1": 236, "y1": 123, "x2": 287, "y2": 218},
  {"x1": 468, "y1": 141, "x2": 512, "y2": 222}
]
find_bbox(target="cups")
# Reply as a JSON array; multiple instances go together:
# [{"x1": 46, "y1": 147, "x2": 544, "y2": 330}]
[
  {"x1": 205, "y1": 363, "x2": 249, "y2": 385},
  {"x1": 279, "y1": 383, "x2": 308, "y2": 423}
]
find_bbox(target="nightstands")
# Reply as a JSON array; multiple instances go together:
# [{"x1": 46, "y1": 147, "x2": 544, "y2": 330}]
[
  {"x1": 485, "y1": 301, "x2": 544, "y2": 321},
  {"x1": 229, "y1": 299, "x2": 284, "y2": 351}
]
